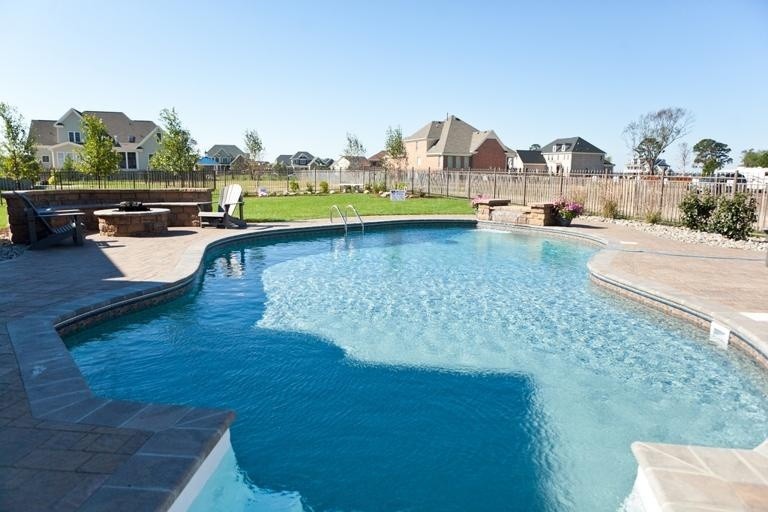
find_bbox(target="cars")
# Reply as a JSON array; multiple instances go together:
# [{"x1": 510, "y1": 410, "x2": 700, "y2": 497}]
[{"x1": 691, "y1": 171, "x2": 746, "y2": 193}]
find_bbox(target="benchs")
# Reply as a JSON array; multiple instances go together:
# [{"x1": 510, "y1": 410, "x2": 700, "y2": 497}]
[
  {"x1": 477, "y1": 199, "x2": 555, "y2": 226},
  {"x1": 24, "y1": 201, "x2": 210, "y2": 236}
]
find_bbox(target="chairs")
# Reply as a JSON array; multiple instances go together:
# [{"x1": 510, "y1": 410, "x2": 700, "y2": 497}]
[
  {"x1": 15, "y1": 190, "x2": 87, "y2": 249},
  {"x1": 198, "y1": 184, "x2": 245, "y2": 229}
]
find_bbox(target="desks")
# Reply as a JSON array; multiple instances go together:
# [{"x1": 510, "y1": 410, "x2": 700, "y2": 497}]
[{"x1": 94, "y1": 208, "x2": 171, "y2": 237}]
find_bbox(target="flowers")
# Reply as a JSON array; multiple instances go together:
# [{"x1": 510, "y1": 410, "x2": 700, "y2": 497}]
[
  {"x1": 472, "y1": 194, "x2": 491, "y2": 209},
  {"x1": 554, "y1": 196, "x2": 583, "y2": 218}
]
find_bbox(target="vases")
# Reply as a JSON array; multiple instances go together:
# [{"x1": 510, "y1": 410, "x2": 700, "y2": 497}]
[
  {"x1": 556, "y1": 217, "x2": 572, "y2": 227},
  {"x1": 473, "y1": 208, "x2": 478, "y2": 216}
]
